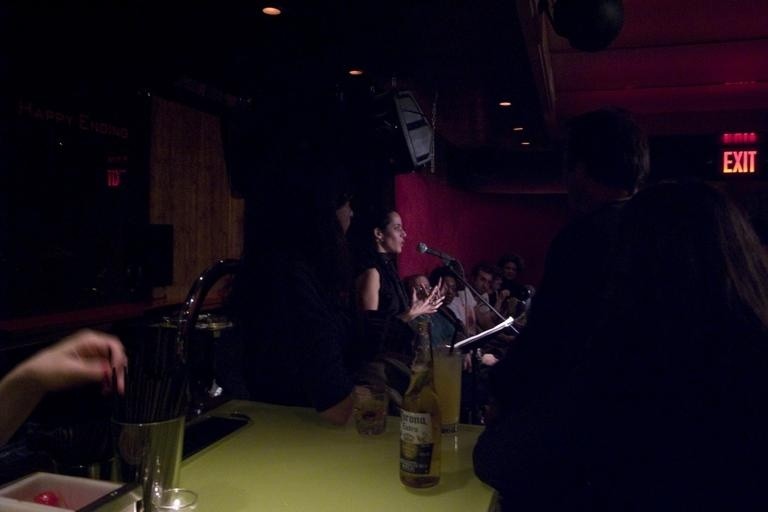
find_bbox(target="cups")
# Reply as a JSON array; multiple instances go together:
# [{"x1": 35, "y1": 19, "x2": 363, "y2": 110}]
[
  {"x1": 104, "y1": 407, "x2": 199, "y2": 512},
  {"x1": 431, "y1": 346, "x2": 464, "y2": 435},
  {"x1": 349, "y1": 383, "x2": 389, "y2": 437}
]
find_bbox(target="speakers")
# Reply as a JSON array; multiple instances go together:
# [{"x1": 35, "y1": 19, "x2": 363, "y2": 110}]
[{"x1": 393, "y1": 90, "x2": 435, "y2": 169}]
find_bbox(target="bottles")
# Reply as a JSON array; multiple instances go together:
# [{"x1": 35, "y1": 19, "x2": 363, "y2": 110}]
[{"x1": 396, "y1": 314, "x2": 442, "y2": 488}]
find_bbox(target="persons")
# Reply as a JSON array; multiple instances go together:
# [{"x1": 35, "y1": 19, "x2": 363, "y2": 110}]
[
  {"x1": 322, "y1": 333, "x2": 442, "y2": 430},
  {"x1": 345, "y1": 206, "x2": 448, "y2": 416},
  {"x1": 472, "y1": 105, "x2": 649, "y2": 512},
  {"x1": 266, "y1": 185, "x2": 357, "y2": 411},
  {"x1": 394, "y1": 252, "x2": 537, "y2": 426},
  {"x1": 585, "y1": 173, "x2": 767, "y2": 512},
  {"x1": 2, "y1": 325, "x2": 131, "y2": 455}
]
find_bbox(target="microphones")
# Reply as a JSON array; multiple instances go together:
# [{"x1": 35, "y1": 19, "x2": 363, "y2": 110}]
[{"x1": 417, "y1": 242, "x2": 456, "y2": 262}]
